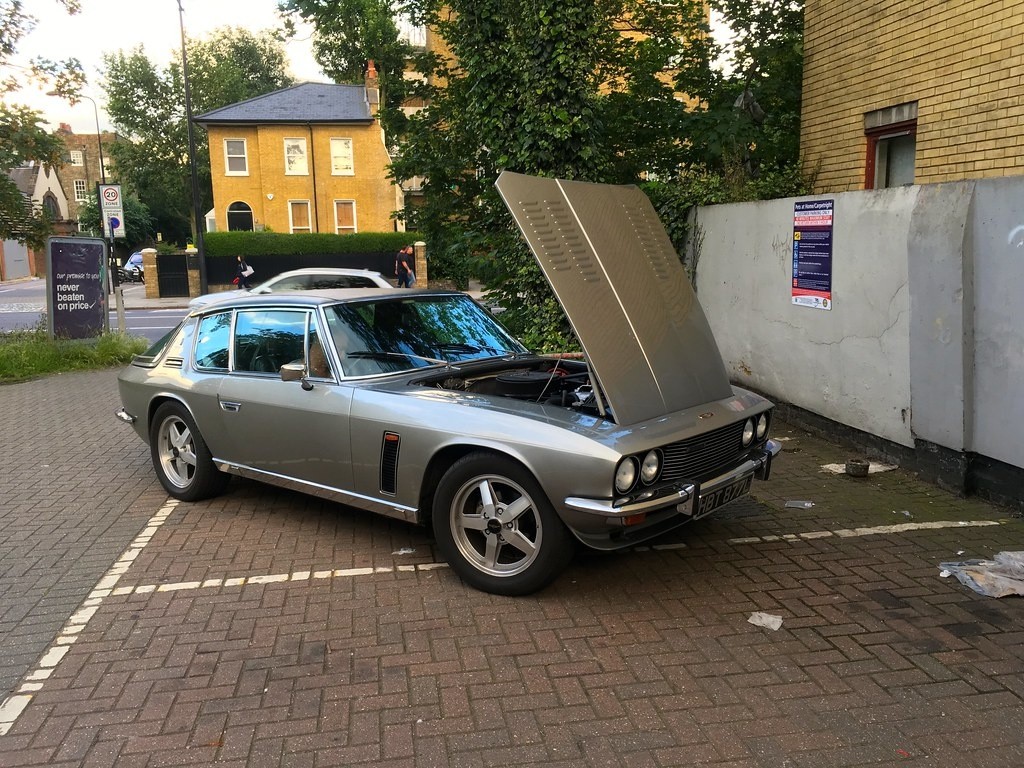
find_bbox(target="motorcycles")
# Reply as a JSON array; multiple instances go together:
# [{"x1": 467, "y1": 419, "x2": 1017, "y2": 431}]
[{"x1": 117, "y1": 260, "x2": 145, "y2": 285}]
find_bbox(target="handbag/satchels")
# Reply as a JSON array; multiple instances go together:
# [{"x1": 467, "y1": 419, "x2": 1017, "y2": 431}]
[
  {"x1": 240, "y1": 262, "x2": 254, "y2": 277},
  {"x1": 233, "y1": 277, "x2": 239, "y2": 284}
]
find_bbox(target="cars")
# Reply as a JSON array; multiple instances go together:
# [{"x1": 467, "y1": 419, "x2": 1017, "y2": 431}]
[
  {"x1": 113, "y1": 171, "x2": 781, "y2": 596},
  {"x1": 124, "y1": 252, "x2": 143, "y2": 272}
]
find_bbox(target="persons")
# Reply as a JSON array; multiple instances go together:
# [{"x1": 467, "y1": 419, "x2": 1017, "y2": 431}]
[
  {"x1": 235, "y1": 255, "x2": 251, "y2": 288},
  {"x1": 395, "y1": 244, "x2": 409, "y2": 288},
  {"x1": 402, "y1": 246, "x2": 414, "y2": 288}
]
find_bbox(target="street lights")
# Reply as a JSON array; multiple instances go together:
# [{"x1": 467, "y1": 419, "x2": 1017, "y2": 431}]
[{"x1": 45, "y1": 91, "x2": 119, "y2": 295}]
